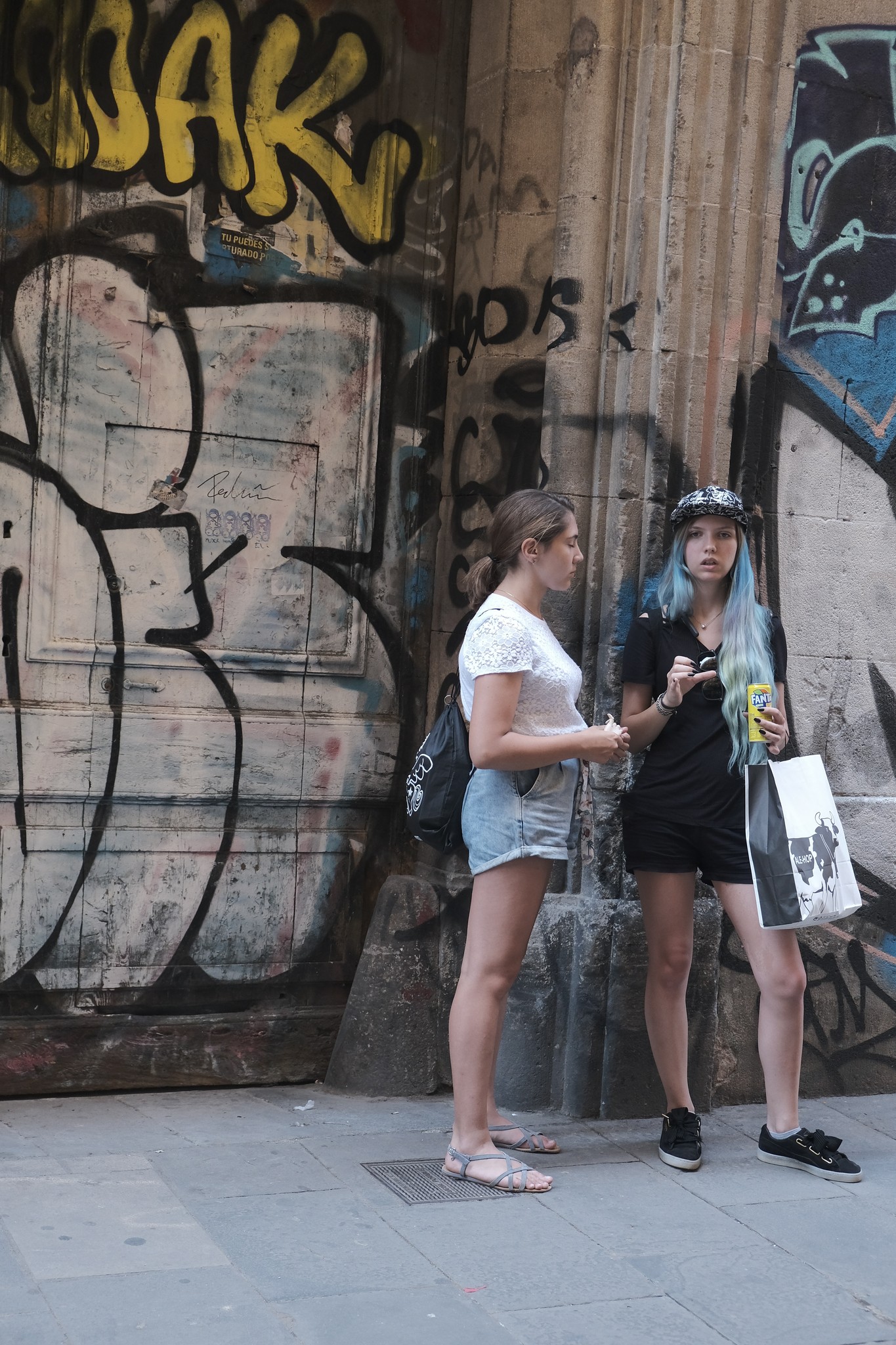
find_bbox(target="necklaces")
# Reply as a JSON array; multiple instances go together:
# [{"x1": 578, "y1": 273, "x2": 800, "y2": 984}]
[{"x1": 690, "y1": 609, "x2": 723, "y2": 628}]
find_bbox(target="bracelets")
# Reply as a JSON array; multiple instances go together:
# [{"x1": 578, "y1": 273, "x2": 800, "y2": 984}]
[{"x1": 655, "y1": 692, "x2": 678, "y2": 717}]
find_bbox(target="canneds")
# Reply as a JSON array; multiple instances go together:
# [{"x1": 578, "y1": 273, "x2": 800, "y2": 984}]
[{"x1": 747, "y1": 683, "x2": 772, "y2": 743}]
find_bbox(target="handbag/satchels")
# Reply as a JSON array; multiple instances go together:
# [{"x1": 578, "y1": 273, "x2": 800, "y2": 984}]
[{"x1": 745, "y1": 754, "x2": 863, "y2": 929}]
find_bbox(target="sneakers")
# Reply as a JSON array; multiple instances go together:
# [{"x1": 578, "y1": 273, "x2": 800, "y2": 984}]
[
  {"x1": 756, "y1": 1123, "x2": 863, "y2": 1183},
  {"x1": 658, "y1": 1107, "x2": 706, "y2": 1170}
]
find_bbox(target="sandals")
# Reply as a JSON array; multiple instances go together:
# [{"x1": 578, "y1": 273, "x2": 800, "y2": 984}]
[
  {"x1": 487, "y1": 1118, "x2": 561, "y2": 1154},
  {"x1": 440, "y1": 1143, "x2": 552, "y2": 1194}
]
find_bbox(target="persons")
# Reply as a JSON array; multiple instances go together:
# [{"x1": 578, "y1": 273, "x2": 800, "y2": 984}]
[
  {"x1": 614, "y1": 485, "x2": 865, "y2": 1184},
  {"x1": 438, "y1": 490, "x2": 633, "y2": 1193}
]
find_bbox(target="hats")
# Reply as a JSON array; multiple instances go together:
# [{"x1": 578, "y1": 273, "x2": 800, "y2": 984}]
[{"x1": 670, "y1": 484, "x2": 748, "y2": 539}]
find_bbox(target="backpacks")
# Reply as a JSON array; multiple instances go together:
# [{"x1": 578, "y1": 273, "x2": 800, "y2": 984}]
[{"x1": 403, "y1": 606, "x2": 506, "y2": 854}]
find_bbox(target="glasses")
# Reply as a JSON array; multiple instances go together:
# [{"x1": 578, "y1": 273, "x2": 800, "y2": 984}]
[{"x1": 697, "y1": 649, "x2": 724, "y2": 702}]
